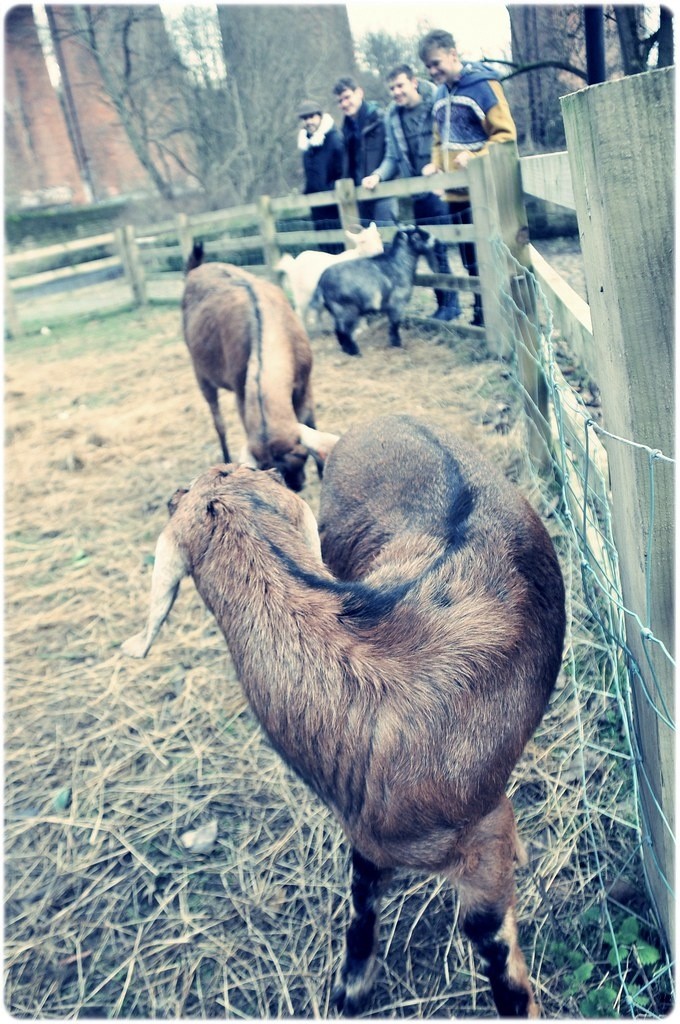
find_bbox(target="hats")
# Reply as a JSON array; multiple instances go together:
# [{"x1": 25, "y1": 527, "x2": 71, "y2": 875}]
[{"x1": 296, "y1": 100, "x2": 322, "y2": 117}]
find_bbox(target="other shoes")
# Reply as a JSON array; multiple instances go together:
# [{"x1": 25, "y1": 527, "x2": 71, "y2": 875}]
[{"x1": 431, "y1": 306, "x2": 460, "y2": 321}]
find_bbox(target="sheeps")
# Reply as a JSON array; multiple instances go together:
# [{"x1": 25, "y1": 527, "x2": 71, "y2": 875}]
[
  {"x1": 271, "y1": 206, "x2": 442, "y2": 358},
  {"x1": 179, "y1": 260, "x2": 346, "y2": 495},
  {"x1": 118, "y1": 407, "x2": 570, "y2": 1020}
]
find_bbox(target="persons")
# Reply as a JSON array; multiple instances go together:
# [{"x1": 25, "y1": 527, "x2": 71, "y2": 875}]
[
  {"x1": 418, "y1": 28, "x2": 517, "y2": 324},
  {"x1": 360, "y1": 63, "x2": 462, "y2": 322},
  {"x1": 332, "y1": 77, "x2": 398, "y2": 253},
  {"x1": 295, "y1": 99, "x2": 347, "y2": 253}
]
